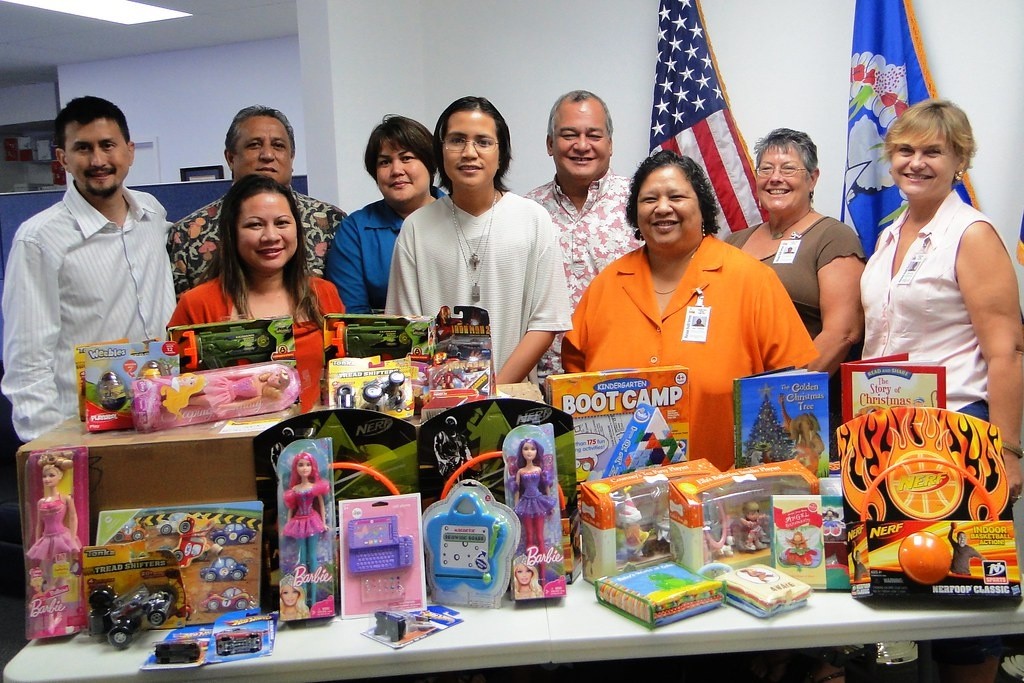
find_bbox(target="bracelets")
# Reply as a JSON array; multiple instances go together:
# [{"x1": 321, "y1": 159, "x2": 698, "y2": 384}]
[{"x1": 1000, "y1": 440, "x2": 1024, "y2": 459}]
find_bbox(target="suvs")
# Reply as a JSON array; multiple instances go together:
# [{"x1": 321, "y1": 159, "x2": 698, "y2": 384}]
[
  {"x1": 109, "y1": 590, "x2": 173, "y2": 650},
  {"x1": 362, "y1": 371, "x2": 405, "y2": 413}
]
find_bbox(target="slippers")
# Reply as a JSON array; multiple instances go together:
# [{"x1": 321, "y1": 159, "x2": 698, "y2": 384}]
[{"x1": 441, "y1": 136, "x2": 501, "y2": 153}]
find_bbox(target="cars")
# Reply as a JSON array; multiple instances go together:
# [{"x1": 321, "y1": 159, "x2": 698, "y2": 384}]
[
  {"x1": 158, "y1": 513, "x2": 195, "y2": 535},
  {"x1": 153, "y1": 643, "x2": 199, "y2": 664},
  {"x1": 198, "y1": 585, "x2": 251, "y2": 610},
  {"x1": 200, "y1": 555, "x2": 249, "y2": 581},
  {"x1": 214, "y1": 628, "x2": 262, "y2": 658},
  {"x1": 210, "y1": 522, "x2": 257, "y2": 545},
  {"x1": 114, "y1": 520, "x2": 149, "y2": 543}
]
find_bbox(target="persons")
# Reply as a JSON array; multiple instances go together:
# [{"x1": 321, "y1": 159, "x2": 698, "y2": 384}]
[
  {"x1": 723, "y1": 129, "x2": 866, "y2": 465},
  {"x1": 281, "y1": 453, "x2": 329, "y2": 614},
  {"x1": 740, "y1": 501, "x2": 770, "y2": 551},
  {"x1": 325, "y1": 113, "x2": 447, "y2": 323},
  {"x1": 564, "y1": 149, "x2": 821, "y2": 473},
  {"x1": 173, "y1": 173, "x2": 347, "y2": 415},
  {"x1": 25, "y1": 450, "x2": 82, "y2": 630},
  {"x1": 947, "y1": 527, "x2": 987, "y2": 576},
  {"x1": 522, "y1": 90, "x2": 649, "y2": 393},
  {"x1": 851, "y1": 548, "x2": 869, "y2": 580},
  {"x1": 144, "y1": 363, "x2": 288, "y2": 421},
  {"x1": 506, "y1": 439, "x2": 555, "y2": 588},
  {"x1": 860, "y1": 97, "x2": 1024, "y2": 523},
  {"x1": 0, "y1": 95, "x2": 175, "y2": 443},
  {"x1": 167, "y1": 105, "x2": 346, "y2": 303}
]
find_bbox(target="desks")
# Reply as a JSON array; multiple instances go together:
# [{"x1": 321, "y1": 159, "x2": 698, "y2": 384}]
[{"x1": 3, "y1": 535, "x2": 1024, "y2": 683}]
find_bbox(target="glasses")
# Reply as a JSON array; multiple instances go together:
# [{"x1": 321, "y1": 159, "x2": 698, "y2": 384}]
[{"x1": 753, "y1": 164, "x2": 807, "y2": 178}]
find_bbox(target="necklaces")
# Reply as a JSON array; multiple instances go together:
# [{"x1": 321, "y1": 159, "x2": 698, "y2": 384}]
[
  {"x1": 447, "y1": 189, "x2": 497, "y2": 302},
  {"x1": 381, "y1": 95, "x2": 575, "y2": 384},
  {"x1": 453, "y1": 200, "x2": 489, "y2": 270},
  {"x1": 653, "y1": 288, "x2": 676, "y2": 294},
  {"x1": 768, "y1": 209, "x2": 811, "y2": 240}
]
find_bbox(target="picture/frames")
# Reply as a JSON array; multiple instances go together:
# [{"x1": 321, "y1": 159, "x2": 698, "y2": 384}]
[{"x1": 180, "y1": 165, "x2": 224, "y2": 181}]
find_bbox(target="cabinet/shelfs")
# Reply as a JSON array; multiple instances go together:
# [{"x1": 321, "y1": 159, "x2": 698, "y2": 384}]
[{"x1": 4, "y1": 135, "x2": 67, "y2": 193}]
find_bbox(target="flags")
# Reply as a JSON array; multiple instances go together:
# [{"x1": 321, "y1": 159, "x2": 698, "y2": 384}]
[
  {"x1": 649, "y1": 0, "x2": 767, "y2": 244},
  {"x1": 839, "y1": 1, "x2": 980, "y2": 264}
]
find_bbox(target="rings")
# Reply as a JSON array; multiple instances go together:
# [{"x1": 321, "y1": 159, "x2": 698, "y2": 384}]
[{"x1": 1012, "y1": 495, "x2": 1023, "y2": 500}]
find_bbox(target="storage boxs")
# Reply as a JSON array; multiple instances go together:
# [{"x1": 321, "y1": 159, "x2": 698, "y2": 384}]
[{"x1": 15, "y1": 366, "x2": 853, "y2": 671}]
